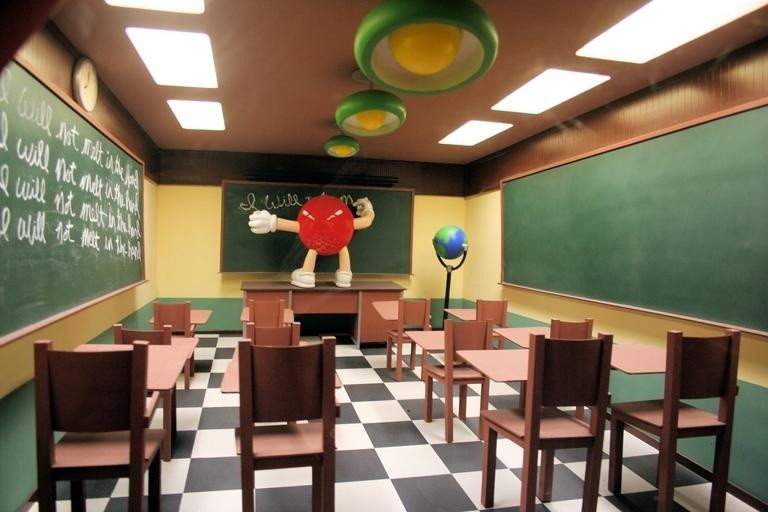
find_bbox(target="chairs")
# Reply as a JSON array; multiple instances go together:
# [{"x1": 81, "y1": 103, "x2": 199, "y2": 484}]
[
  {"x1": 474, "y1": 299, "x2": 510, "y2": 351},
  {"x1": 480, "y1": 331, "x2": 612, "y2": 511},
  {"x1": 33, "y1": 340, "x2": 156, "y2": 512},
  {"x1": 114, "y1": 323, "x2": 177, "y2": 442},
  {"x1": 236, "y1": 335, "x2": 336, "y2": 511},
  {"x1": 385, "y1": 296, "x2": 430, "y2": 381},
  {"x1": 424, "y1": 319, "x2": 493, "y2": 441},
  {"x1": 243, "y1": 320, "x2": 303, "y2": 346},
  {"x1": 248, "y1": 299, "x2": 284, "y2": 327},
  {"x1": 609, "y1": 328, "x2": 741, "y2": 512},
  {"x1": 549, "y1": 318, "x2": 587, "y2": 343},
  {"x1": 151, "y1": 301, "x2": 194, "y2": 376}
]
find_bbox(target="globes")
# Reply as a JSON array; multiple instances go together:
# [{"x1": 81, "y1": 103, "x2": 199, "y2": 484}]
[{"x1": 432, "y1": 224, "x2": 468, "y2": 330}]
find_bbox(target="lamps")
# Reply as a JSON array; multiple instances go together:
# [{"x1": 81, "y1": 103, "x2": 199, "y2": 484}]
[
  {"x1": 334, "y1": 91, "x2": 406, "y2": 138},
  {"x1": 325, "y1": 137, "x2": 362, "y2": 159},
  {"x1": 353, "y1": 1, "x2": 499, "y2": 97}
]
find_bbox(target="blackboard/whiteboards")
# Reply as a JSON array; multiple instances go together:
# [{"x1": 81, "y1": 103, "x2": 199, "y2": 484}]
[
  {"x1": 0, "y1": 37, "x2": 150, "y2": 350},
  {"x1": 219, "y1": 178, "x2": 416, "y2": 279},
  {"x1": 496, "y1": 95, "x2": 768, "y2": 343}
]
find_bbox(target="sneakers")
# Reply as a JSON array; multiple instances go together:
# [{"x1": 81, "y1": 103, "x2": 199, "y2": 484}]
[
  {"x1": 290, "y1": 268, "x2": 316, "y2": 288},
  {"x1": 333, "y1": 269, "x2": 352, "y2": 288}
]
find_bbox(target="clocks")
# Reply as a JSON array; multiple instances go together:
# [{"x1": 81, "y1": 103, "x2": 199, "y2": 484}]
[{"x1": 73, "y1": 57, "x2": 99, "y2": 112}]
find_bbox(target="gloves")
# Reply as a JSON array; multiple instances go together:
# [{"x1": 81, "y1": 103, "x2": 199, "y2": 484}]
[
  {"x1": 352, "y1": 197, "x2": 375, "y2": 221},
  {"x1": 248, "y1": 210, "x2": 276, "y2": 235}
]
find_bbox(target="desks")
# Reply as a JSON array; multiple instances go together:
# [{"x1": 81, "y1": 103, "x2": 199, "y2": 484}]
[
  {"x1": 70, "y1": 343, "x2": 190, "y2": 461},
  {"x1": 604, "y1": 342, "x2": 680, "y2": 377},
  {"x1": 167, "y1": 338, "x2": 198, "y2": 390},
  {"x1": 373, "y1": 300, "x2": 399, "y2": 320},
  {"x1": 492, "y1": 326, "x2": 553, "y2": 349},
  {"x1": 221, "y1": 356, "x2": 343, "y2": 424},
  {"x1": 456, "y1": 349, "x2": 529, "y2": 409},
  {"x1": 440, "y1": 308, "x2": 478, "y2": 322},
  {"x1": 148, "y1": 309, "x2": 212, "y2": 338},
  {"x1": 241, "y1": 275, "x2": 405, "y2": 350},
  {"x1": 405, "y1": 331, "x2": 446, "y2": 351}
]
